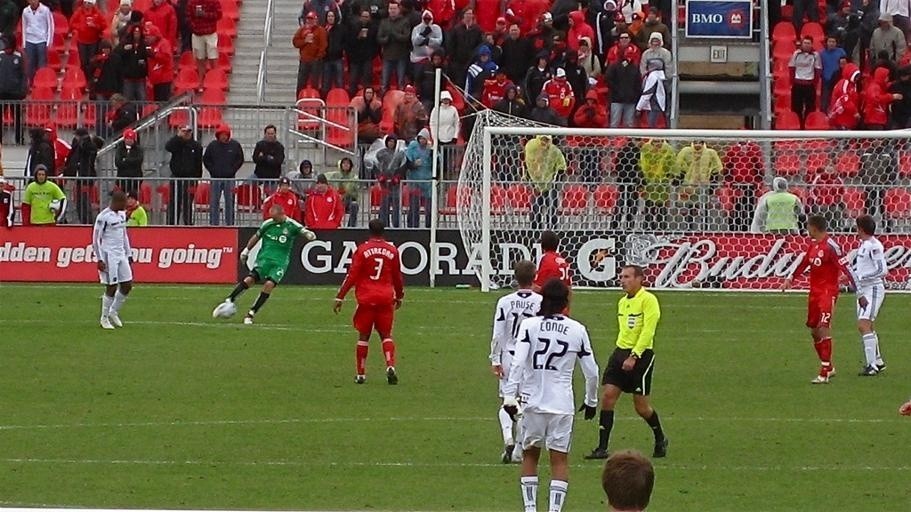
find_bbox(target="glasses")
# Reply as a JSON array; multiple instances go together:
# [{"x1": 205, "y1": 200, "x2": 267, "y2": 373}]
[{"x1": 620, "y1": 36, "x2": 629, "y2": 39}]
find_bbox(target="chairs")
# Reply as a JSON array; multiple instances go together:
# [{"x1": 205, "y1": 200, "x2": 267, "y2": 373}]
[
  {"x1": 325, "y1": 88, "x2": 349, "y2": 121},
  {"x1": 773, "y1": 59, "x2": 794, "y2": 78},
  {"x1": 3, "y1": 108, "x2": 14, "y2": 126},
  {"x1": 775, "y1": 155, "x2": 800, "y2": 174},
  {"x1": 775, "y1": 111, "x2": 800, "y2": 130},
  {"x1": 556, "y1": 185, "x2": 589, "y2": 216},
  {"x1": 883, "y1": 188, "x2": 911, "y2": 219},
  {"x1": 807, "y1": 154, "x2": 828, "y2": 172},
  {"x1": 176, "y1": 51, "x2": 199, "y2": 71},
  {"x1": 298, "y1": 111, "x2": 320, "y2": 130},
  {"x1": 716, "y1": 187, "x2": 735, "y2": 210},
  {"x1": 236, "y1": 186, "x2": 260, "y2": 212},
  {"x1": 773, "y1": 75, "x2": 793, "y2": 94},
  {"x1": 508, "y1": 187, "x2": 534, "y2": 215},
  {"x1": 401, "y1": 186, "x2": 411, "y2": 207},
  {"x1": 440, "y1": 186, "x2": 472, "y2": 215},
  {"x1": 489, "y1": 186, "x2": 506, "y2": 216},
  {"x1": 772, "y1": 21, "x2": 797, "y2": 40},
  {"x1": 74, "y1": 186, "x2": 99, "y2": 204},
  {"x1": 203, "y1": 69, "x2": 229, "y2": 91},
  {"x1": 897, "y1": 154, "x2": 911, "y2": 178},
  {"x1": 217, "y1": 15, "x2": 236, "y2": 38},
  {"x1": 328, "y1": 126, "x2": 353, "y2": 147},
  {"x1": 803, "y1": 141, "x2": 828, "y2": 151},
  {"x1": 197, "y1": 109, "x2": 222, "y2": 128},
  {"x1": 63, "y1": 68, "x2": 86, "y2": 87},
  {"x1": 222, "y1": 0, "x2": 240, "y2": 21},
  {"x1": 31, "y1": 85, "x2": 54, "y2": 101},
  {"x1": 175, "y1": 69, "x2": 200, "y2": 92},
  {"x1": 53, "y1": 33, "x2": 66, "y2": 53},
  {"x1": 82, "y1": 104, "x2": 96, "y2": 128},
  {"x1": 595, "y1": 188, "x2": 618, "y2": 216},
  {"x1": 156, "y1": 185, "x2": 168, "y2": 205},
  {"x1": 168, "y1": 106, "x2": 189, "y2": 130},
  {"x1": 48, "y1": 48, "x2": 62, "y2": 72},
  {"x1": 844, "y1": 189, "x2": 865, "y2": 218},
  {"x1": 141, "y1": 104, "x2": 160, "y2": 121},
  {"x1": 54, "y1": 104, "x2": 76, "y2": 128},
  {"x1": 774, "y1": 94, "x2": 792, "y2": 114},
  {"x1": 805, "y1": 111, "x2": 828, "y2": 130},
  {"x1": 139, "y1": 184, "x2": 151, "y2": 205},
  {"x1": 773, "y1": 39, "x2": 795, "y2": 58},
  {"x1": 193, "y1": 183, "x2": 209, "y2": 205},
  {"x1": 837, "y1": 156, "x2": 860, "y2": 177},
  {"x1": 217, "y1": 32, "x2": 235, "y2": 56},
  {"x1": 61, "y1": 85, "x2": 82, "y2": 101},
  {"x1": 33, "y1": 68, "x2": 57, "y2": 87},
  {"x1": 54, "y1": 14, "x2": 68, "y2": 34},
  {"x1": 776, "y1": 141, "x2": 802, "y2": 152},
  {"x1": 206, "y1": 52, "x2": 232, "y2": 73},
  {"x1": 298, "y1": 88, "x2": 320, "y2": 112},
  {"x1": 370, "y1": 185, "x2": 385, "y2": 206},
  {"x1": 203, "y1": 87, "x2": 225, "y2": 109},
  {"x1": 800, "y1": 22, "x2": 824, "y2": 41},
  {"x1": 24, "y1": 103, "x2": 50, "y2": 126},
  {"x1": 788, "y1": 188, "x2": 807, "y2": 207},
  {"x1": 379, "y1": 90, "x2": 405, "y2": 132}
]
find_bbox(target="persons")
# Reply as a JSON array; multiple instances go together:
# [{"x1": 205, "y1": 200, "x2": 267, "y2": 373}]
[
  {"x1": 494, "y1": 78, "x2": 528, "y2": 126},
  {"x1": 353, "y1": 84, "x2": 383, "y2": 143},
  {"x1": 898, "y1": 399, "x2": 911, "y2": 417},
  {"x1": 261, "y1": 177, "x2": 302, "y2": 223},
  {"x1": 109, "y1": 1, "x2": 143, "y2": 42},
  {"x1": 348, "y1": 11, "x2": 381, "y2": 98},
  {"x1": 21, "y1": 0, "x2": 55, "y2": 87},
  {"x1": 847, "y1": 214, "x2": 888, "y2": 376},
  {"x1": 816, "y1": 36, "x2": 849, "y2": 112},
  {"x1": 332, "y1": 220, "x2": 405, "y2": 386},
  {"x1": 64, "y1": 128, "x2": 98, "y2": 224},
  {"x1": 498, "y1": 23, "x2": 535, "y2": 85},
  {"x1": 376, "y1": 1, "x2": 412, "y2": 97},
  {"x1": 224, "y1": 203, "x2": 316, "y2": 325},
  {"x1": 412, "y1": 8, "x2": 444, "y2": 87},
  {"x1": 790, "y1": 0, "x2": 821, "y2": 37},
  {"x1": 665, "y1": 138, "x2": 723, "y2": 229},
  {"x1": 608, "y1": 136, "x2": 641, "y2": 230},
  {"x1": 304, "y1": 173, "x2": 346, "y2": 230},
  {"x1": 583, "y1": 265, "x2": 669, "y2": 460},
  {"x1": 114, "y1": 24, "x2": 148, "y2": 102},
  {"x1": 429, "y1": 91, "x2": 461, "y2": 179},
  {"x1": 574, "y1": 35, "x2": 601, "y2": 81},
  {"x1": 826, "y1": 62, "x2": 863, "y2": 130},
  {"x1": 456, "y1": 91, "x2": 486, "y2": 149},
  {"x1": 542, "y1": 67, "x2": 575, "y2": 128},
  {"x1": 889, "y1": 65, "x2": 911, "y2": 130},
  {"x1": 491, "y1": 16, "x2": 512, "y2": 48},
  {"x1": 414, "y1": 48, "x2": 447, "y2": 110},
  {"x1": 489, "y1": 259, "x2": 544, "y2": 465},
  {"x1": 749, "y1": 176, "x2": 808, "y2": 233},
  {"x1": 186, "y1": 0, "x2": 223, "y2": 94},
  {"x1": 290, "y1": 159, "x2": 316, "y2": 201},
  {"x1": 525, "y1": 89, "x2": 558, "y2": 125},
  {"x1": 447, "y1": 6, "x2": 483, "y2": 88},
  {"x1": 555, "y1": 49, "x2": 591, "y2": 100},
  {"x1": 375, "y1": 135, "x2": 411, "y2": 227},
  {"x1": 870, "y1": 50, "x2": 898, "y2": 75},
  {"x1": 808, "y1": 163, "x2": 846, "y2": 231},
  {"x1": 522, "y1": 49, "x2": 554, "y2": 102},
  {"x1": 604, "y1": 46, "x2": 643, "y2": 127},
  {"x1": 600, "y1": 450, "x2": 655, "y2": 512},
  {"x1": 502, "y1": 276, "x2": 600, "y2": 512},
  {"x1": 321, "y1": 10, "x2": 347, "y2": 101},
  {"x1": 641, "y1": 139, "x2": 678, "y2": 230},
  {"x1": 856, "y1": 138, "x2": 901, "y2": 236},
  {"x1": 91, "y1": 190, "x2": 135, "y2": 331},
  {"x1": 67, "y1": 0, "x2": 106, "y2": 92},
  {"x1": 104, "y1": 92, "x2": 138, "y2": 137},
  {"x1": 87, "y1": 40, "x2": 117, "y2": 137},
  {"x1": 142, "y1": 24, "x2": 174, "y2": 102},
  {"x1": 23, "y1": 127, "x2": 57, "y2": 184},
  {"x1": 523, "y1": 135, "x2": 567, "y2": 228},
  {"x1": 868, "y1": 12, "x2": 907, "y2": 68},
  {"x1": 202, "y1": 123, "x2": 245, "y2": 227},
  {"x1": 863, "y1": 67, "x2": 903, "y2": 130},
  {"x1": 637, "y1": 7, "x2": 671, "y2": 54},
  {"x1": 0, "y1": 30, "x2": 32, "y2": 146},
  {"x1": 328, "y1": 157, "x2": 360, "y2": 228},
  {"x1": 566, "y1": 10, "x2": 596, "y2": 52},
  {"x1": 826, "y1": 57, "x2": 852, "y2": 104},
  {"x1": 878, "y1": 0, "x2": 910, "y2": 35},
  {"x1": 481, "y1": 67, "x2": 515, "y2": 112},
  {"x1": 638, "y1": 31, "x2": 676, "y2": 128},
  {"x1": 243, "y1": 124, "x2": 285, "y2": 200},
  {"x1": 0, "y1": 181, "x2": 19, "y2": 228},
  {"x1": 628, "y1": 12, "x2": 644, "y2": 36},
  {"x1": 292, "y1": 10, "x2": 329, "y2": 101},
  {"x1": 22, "y1": 163, "x2": 68, "y2": 227},
  {"x1": 845, "y1": 14, "x2": 866, "y2": 68},
  {"x1": 406, "y1": 128, "x2": 440, "y2": 227},
  {"x1": 594, "y1": 0, "x2": 623, "y2": 29},
  {"x1": 461, "y1": 42, "x2": 498, "y2": 137},
  {"x1": 788, "y1": 35, "x2": 824, "y2": 130},
  {"x1": 604, "y1": 11, "x2": 630, "y2": 40},
  {"x1": 779, "y1": 216, "x2": 869, "y2": 384},
  {"x1": 722, "y1": 141, "x2": 766, "y2": 232},
  {"x1": 607, "y1": 30, "x2": 641, "y2": 66},
  {"x1": 123, "y1": 191, "x2": 148, "y2": 227},
  {"x1": 393, "y1": 83, "x2": 430, "y2": 143},
  {"x1": 824, "y1": 0, "x2": 853, "y2": 48},
  {"x1": 573, "y1": 90, "x2": 610, "y2": 130},
  {"x1": 164, "y1": 122, "x2": 204, "y2": 225},
  {"x1": 114, "y1": 128, "x2": 143, "y2": 202},
  {"x1": 530, "y1": 231, "x2": 573, "y2": 320}
]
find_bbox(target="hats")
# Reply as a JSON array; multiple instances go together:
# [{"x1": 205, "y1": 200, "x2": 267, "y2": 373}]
[
  {"x1": 123, "y1": 129, "x2": 137, "y2": 139},
  {"x1": 120, "y1": 0, "x2": 131, "y2": 6},
  {"x1": 131, "y1": 10, "x2": 143, "y2": 22},
  {"x1": 614, "y1": 15, "x2": 625, "y2": 21},
  {"x1": 317, "y1": 173, "x2": 327, "y2": 184},
  {"x1": 555, "y1": 66, "x2": 566, "y2": 77},
  {"x1": 178, "y1": 123, "x2": 192, "y2": 133},
  {"x1": 279, "y1": 177, "x2": 291, "y2": 187}
]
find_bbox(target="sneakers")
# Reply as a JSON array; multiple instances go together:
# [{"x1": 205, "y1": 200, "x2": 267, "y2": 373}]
[
  {"x1": 811, "y1": 375, "x2": 828, "y2": 384},
  {"x1": 858, "y1": 364, "x2": 879, "y2": 376},
  {"x1": 877, "y1": 357, "x2": 886, "y2": 371},
  {"x1": 243, "y1": 312, "x2": 253, "y2": 325},
  {"x1": 108, "y1": 310, "x2": 124, "y2": 328},
  {"x1": 511, "y1": 450, "x2": 522, "y2": 463},
  {"x1": 501, "y1": 438, "x2": 514, "y2": 462},
  {"x1": 584, "y1": 447, "x2": 608, "y2": 458},
  {"x1": 829, "y1": 368, "x2": 836, "y2": 377},
  {"x1": 353, "y1": 374, "x2": 366, "y2": 384},
  {"x1": 99, "y1": 317, "x2": 114, "y2": 330},
  {"x1": 387, "y1": 366, "x2": 398, "y2": 384},
  {"x1": 653, "y1": 438, "x2": 669, "y2": 457}
]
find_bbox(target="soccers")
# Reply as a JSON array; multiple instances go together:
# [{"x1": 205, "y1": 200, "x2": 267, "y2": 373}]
[{"x1": 212, "y1": 302, "x2": 237, "y2": 320}]
